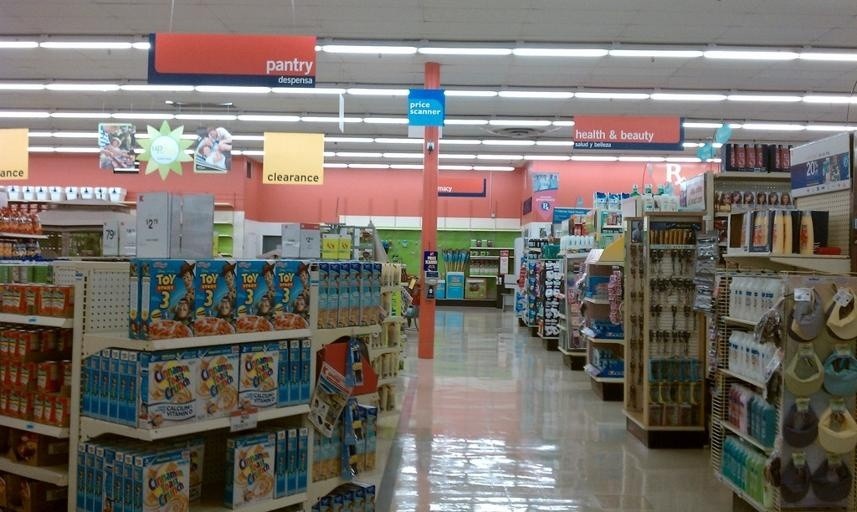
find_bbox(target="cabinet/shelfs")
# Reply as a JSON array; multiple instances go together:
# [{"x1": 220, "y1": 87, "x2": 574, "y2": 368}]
[{"x1": 468, "y1": 247, "x2": 515, "y2": 277}]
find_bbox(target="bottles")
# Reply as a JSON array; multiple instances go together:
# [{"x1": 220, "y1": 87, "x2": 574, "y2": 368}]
[
  {"x1": 724, "y1": 270, "x2": 782, "y2": 503},
  {"x1": 0, "y1": 202, "x2": 101, "y2": 257}
]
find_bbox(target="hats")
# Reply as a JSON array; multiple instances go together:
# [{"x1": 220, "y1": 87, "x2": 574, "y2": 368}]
[{"x1": 781, "y1": 285, "x2": 857, "y2": 501}]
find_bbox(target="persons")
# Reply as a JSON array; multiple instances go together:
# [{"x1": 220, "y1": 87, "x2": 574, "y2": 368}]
[
  {"x1": 757, "y1": 192, "x2": 767, "y2": 205},
  {"x1": 780, "y1": 192, "x2": 791, "y2": 205},
  {"x1": 720, "y1": 191, "x2": 732, "y2": 204},
  {"x1": 197, "y1": 142, "x2": 231, "y2": 170},
  {"x1": 105, "y1": 123, "x2": 136, "y2": 155},
  {"x1": 207, "y1": 126, "x2": 233, "y2": 158},
  {"x1": 103, "y1": 137, "x2": 134, "y2": 167},
  {"x1": 743, "y1": 191, "x2": 754, "y2": 204},
  {"x1": 732, "y1": 191, "x2": 742, "y2": 204},
  {"x1": 768, "y1": 192, "x2": 779, "y2": 206}
]
